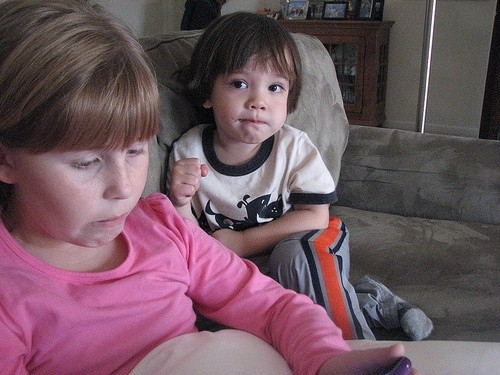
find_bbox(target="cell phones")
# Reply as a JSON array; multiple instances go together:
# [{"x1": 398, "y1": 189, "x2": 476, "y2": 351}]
[{"x1": 377, "y1": 357, "x2": 413, "y2": 375}]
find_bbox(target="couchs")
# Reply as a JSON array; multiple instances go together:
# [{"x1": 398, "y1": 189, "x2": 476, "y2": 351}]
[{"x1": 136, "y1": 25, "x2": 500, "y2": 342}]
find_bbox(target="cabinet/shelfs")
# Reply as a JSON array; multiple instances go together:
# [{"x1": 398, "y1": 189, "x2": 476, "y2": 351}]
[{"x1": 279, "y1": 20, "x2": 396, "y2": 127}]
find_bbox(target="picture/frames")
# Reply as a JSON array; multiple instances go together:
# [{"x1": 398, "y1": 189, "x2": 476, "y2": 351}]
[{"x1": 280, "y1": 0, "x2": 385, "y2": 21}]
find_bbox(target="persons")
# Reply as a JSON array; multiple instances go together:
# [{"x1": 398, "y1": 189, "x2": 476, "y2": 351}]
[
  {"x1": 0, "y1": 0, "x2": 405, "y2": 375},
  {"x1": 167, "y1": 11, "x2": 432, "y2": 342}
]
